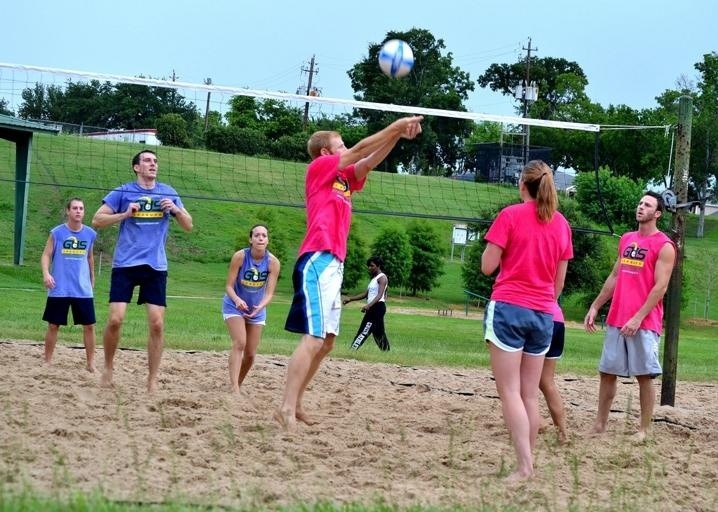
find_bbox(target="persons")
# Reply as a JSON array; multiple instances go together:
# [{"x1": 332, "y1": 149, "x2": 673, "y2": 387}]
[
  {"x1": 41, "y1": 198, "x2": 100, "y2": 375},
  {"x1": 273, "y1": 115, "x2": 424, "y2": 432},
  {"x1": 584, "y1": 191, "x2": 677, "y2": 444},
  {"x1": 482, "y1": 160, "x2": 574, "y2": 486},
  {"x1": 223, "y1": 224, "x2": 280, "y2": 396},
  {"x1": 343, "y1": 256, "x2": 390, "y2": 351},
  {"x1": 91, "y1": 150, "x2": 192, "y2": 393}
]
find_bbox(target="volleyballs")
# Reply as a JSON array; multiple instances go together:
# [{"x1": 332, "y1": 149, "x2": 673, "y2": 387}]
[{"x1": 378, "y1": 39, "x2": 414, "y2": 80}]
